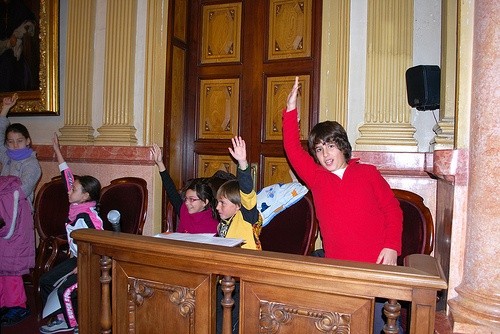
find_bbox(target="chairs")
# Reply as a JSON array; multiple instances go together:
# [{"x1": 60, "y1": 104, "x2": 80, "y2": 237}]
[
  {"x1": 22, "y1": 175, "x2": 149, "y2": 320},
  {"x1": 257, "y1": 189, "x2": 317, "y2": 257},
  {"x1": 378, "y1": 188, "x2": 435, "y2": 305}
]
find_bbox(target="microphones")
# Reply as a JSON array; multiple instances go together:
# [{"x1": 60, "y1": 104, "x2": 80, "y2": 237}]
[{"x1": 107, "y1": 210, "x2": 121, "y2": 233}]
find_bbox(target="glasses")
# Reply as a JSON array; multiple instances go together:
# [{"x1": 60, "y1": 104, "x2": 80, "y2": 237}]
[{"x1": 184, "y1": 197, "x2": 201, "y2": 202}]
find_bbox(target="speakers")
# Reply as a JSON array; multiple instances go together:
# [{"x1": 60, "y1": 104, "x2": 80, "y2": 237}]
[{"x1": 404, "y1": 65, "x2": 442, "y2": 111}]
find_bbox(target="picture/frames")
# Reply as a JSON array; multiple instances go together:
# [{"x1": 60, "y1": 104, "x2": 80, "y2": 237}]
[{"x1": 0, "y1": 0, "x2": 60, "y2": 116}]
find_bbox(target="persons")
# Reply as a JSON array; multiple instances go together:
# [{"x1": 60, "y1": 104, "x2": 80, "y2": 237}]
[
  {"x1": 39, "y1": 133, "x2": 104, "y2": 334},
  {"x1": 0, "y1": 94, "x2": 42, "y2": 328},
  {"x1": 282, "y1": 76, "x2": 405, "y2": 334},
  {"x1": 216, "y1": 136, "x2": 263, "y2": 334},
  {"x1": 150, "y1": 143, "x2": 219, "y2": 234}
]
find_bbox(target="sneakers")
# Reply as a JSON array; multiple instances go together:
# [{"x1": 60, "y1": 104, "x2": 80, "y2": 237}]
[
  {"x1": 40, "y1": 319, "x2": 75, "y2": 334},
  {"x1": 0, "y1": 302, "x2": 31, "y2": 328}
]
